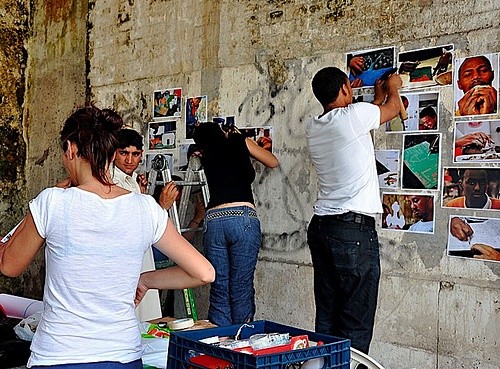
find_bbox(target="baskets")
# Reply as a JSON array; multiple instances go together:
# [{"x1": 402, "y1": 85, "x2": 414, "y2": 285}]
[{"x1": 167, "y1": 321, "x2": 351, "y2": 369}]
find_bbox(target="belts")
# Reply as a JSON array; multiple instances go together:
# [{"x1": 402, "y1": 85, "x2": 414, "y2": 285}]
[{"x1": 206, "y1": 210, "x2": 257, "y2": 222}]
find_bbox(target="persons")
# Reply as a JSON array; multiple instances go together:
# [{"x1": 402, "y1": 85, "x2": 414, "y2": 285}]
[
  {"x1": 454, "y1": 122, "x2": 495, "y2": 162},
  {"x1": 405, "y1": 195, "x2": 434, "y2": 232},
  {"x1": 106, "y1": 129, "x2": 181, "y2": 322},
  {"x1": 442, "y1": 169, "x2": 500, "y2": 210},
  {"x1": 454, "y1": 55, "x2": 497, "y2": 117},
  {"x1": 419, "y1": 106, "x2": 437, "y2": 130},
  {"x1": 0, "y1": 105, "x2": 217, "y2": 369},
  {"x1": 347, "y1": 54, "x2": 393, "y2": 87},
  {"x1": 151, "y1": 173, "x2": 207, "y2": 317},
  {"x1": 191, "y1": 121, "x2": 279, "y2": 328},
  {"x1": 450, "y1": 217, "x2": 500, "y2": 262},
  {"x1": 307, "y1": 66, "x2": 403, "y2": 369}
]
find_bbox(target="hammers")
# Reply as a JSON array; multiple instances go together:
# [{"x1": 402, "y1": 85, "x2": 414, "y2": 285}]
[{"x1": 376, "y1": 67, "x2": 409, "y2": 121}]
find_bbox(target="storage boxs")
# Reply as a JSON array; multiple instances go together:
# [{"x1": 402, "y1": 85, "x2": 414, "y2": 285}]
[{"x1": 165, "y1": 319, "x2": 351, "y2": 369}]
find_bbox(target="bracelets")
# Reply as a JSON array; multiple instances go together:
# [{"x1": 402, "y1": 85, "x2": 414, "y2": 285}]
[{"x1": 191, "y1": 220, "x2": 199, "y2": 226}]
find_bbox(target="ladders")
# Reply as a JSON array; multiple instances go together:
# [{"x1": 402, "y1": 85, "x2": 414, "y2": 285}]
[{"x1": 146, "y1": 150, "x2": 215, "y2": 323}]
